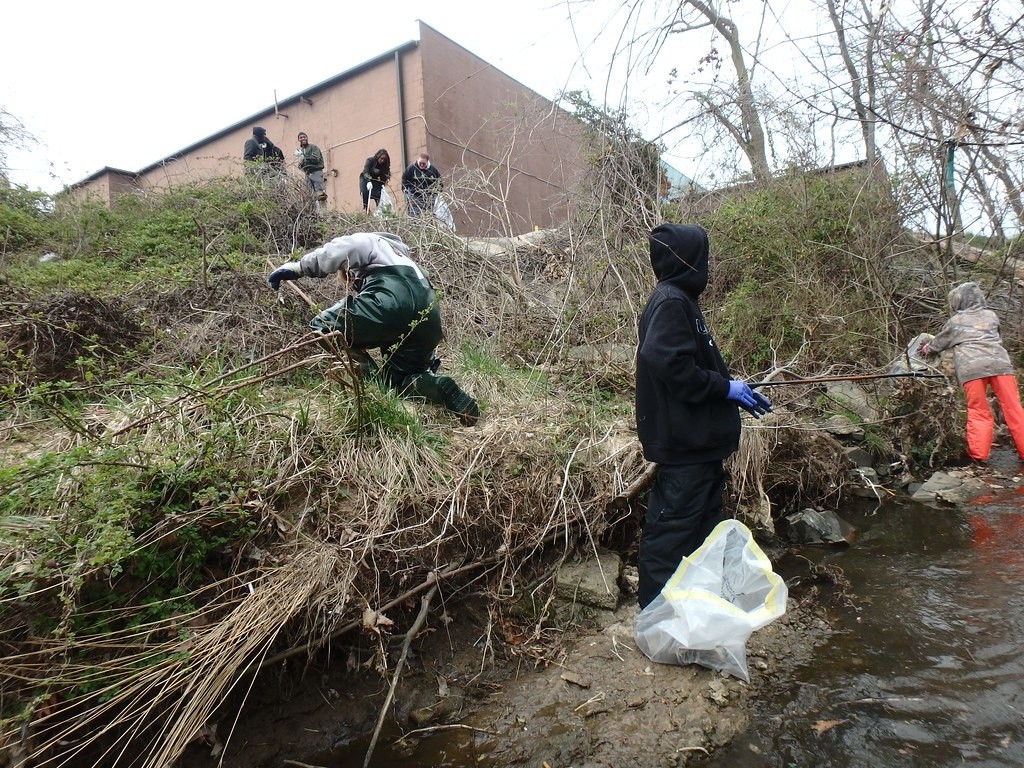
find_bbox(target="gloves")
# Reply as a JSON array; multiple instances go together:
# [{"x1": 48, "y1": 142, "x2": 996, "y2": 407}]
[
  {"x1": 293, "y1": 150, "x2": 303, "y2": 157},
  {"x1": 726, "y1": 390, "x2": 773, "y2": 419},
  {"x1": 366, "y1": 182, "x2": 373, "y2": 190},
  {"x1": 268, "y1": 260, "x2": 305, "y2": 290},
  {"x1": 725, "y1": 380, "x2": 757, "y2": 408}
]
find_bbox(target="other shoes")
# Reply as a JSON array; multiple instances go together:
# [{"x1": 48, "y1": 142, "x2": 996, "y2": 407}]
[
  {"x1": 972, "y1": 460, "x2": 987, "y2": 470},
  {"x1": 434, "y1": 376, "x2": 478, "y2": 427}
]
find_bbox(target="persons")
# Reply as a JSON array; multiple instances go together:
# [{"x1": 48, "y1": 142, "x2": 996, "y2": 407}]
[
  {"x1": 635, "y1": 220, "x2": 774, "y2": 612},
  {"x1": 918, "y1": 280, "x2": 1024, "y2": 466},
  {"x1": 292, "y1": 131, "x2": 328, "y2": 212},
  {"x1": 244, "y1": 127, "x2": 287, "y2": 183},
  {"x1": 400, "y1": 155, "x2": 444, "y2": 224},
  {"x1": 268, "y1": 230, "x2": 479, "y2": 428},
  {"x1": 359, "y1": 150, "x2": 392, "y2": 214}
]
